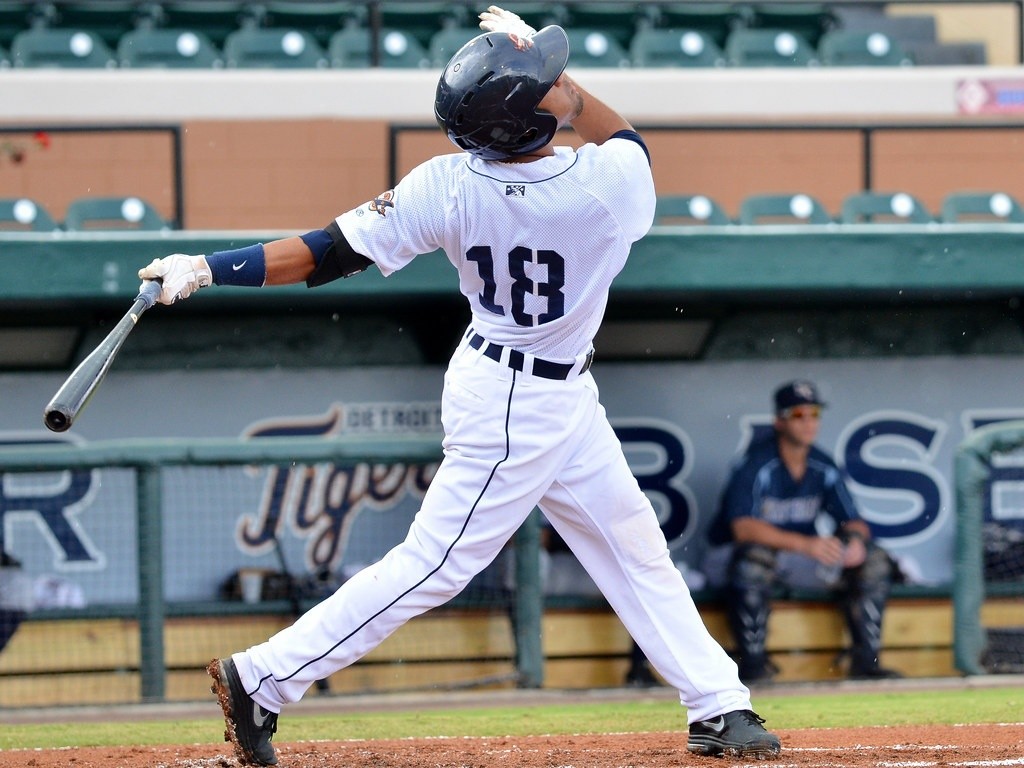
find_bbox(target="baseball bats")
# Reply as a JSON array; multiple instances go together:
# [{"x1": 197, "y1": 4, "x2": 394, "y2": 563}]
[{"x1": 43, "y1": 277, "x2": 163, "y2": 433}]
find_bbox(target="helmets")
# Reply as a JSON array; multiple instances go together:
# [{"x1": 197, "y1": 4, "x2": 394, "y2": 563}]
[{"x1": 434, "y1": 26, "x2": 570, "y2": 160}]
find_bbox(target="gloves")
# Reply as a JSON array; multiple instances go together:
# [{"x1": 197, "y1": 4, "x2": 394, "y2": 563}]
[
  {"x1": 477, "y1": 5, "x2": 538, "y2": 38},
  {"x1": 139, "y1": 253, "x2": 212, "y2": 304}
]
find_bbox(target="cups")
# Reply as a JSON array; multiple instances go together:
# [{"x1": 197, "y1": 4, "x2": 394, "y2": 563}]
[{"x1": 240, "y1": 571, "x2": 263, "y2": 605}]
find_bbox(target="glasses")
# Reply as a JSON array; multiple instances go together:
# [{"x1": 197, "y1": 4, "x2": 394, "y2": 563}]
[{"x1": 789, "y1": 409, "x2": 818, "y2": 420}]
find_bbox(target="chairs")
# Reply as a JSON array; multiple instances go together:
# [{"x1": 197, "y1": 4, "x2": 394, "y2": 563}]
[{"x1": 0, "y1": 0, "x2": 1024, "y2": 234}]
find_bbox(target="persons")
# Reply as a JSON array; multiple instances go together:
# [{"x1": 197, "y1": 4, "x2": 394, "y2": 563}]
[
  {"x1": 704, "y1": 381, "x2": 904, "y2": 682},
  {"x1": 540, "y1": 522, "x2": 662, "y2": 688},
  {"x1": 139, "y1": 6, "x2": 781, "y2": 759}
]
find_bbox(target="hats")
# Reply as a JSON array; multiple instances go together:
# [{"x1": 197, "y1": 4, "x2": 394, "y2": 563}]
[{"x1": 775, "y1": 382, "x2": 825, "y2": 409}]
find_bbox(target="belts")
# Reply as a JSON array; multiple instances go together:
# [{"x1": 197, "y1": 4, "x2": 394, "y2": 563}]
[{"x1": 466, "y1": 329, "x2": 592, "y2": 380}]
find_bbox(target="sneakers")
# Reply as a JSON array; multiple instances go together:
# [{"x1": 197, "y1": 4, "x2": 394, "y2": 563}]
[
  {"x1": 207, "y1": 657, "x2": 279, "y2": 765},
  {"x1": 687, "y1": 710, "x2": 781, "y2": 759}
]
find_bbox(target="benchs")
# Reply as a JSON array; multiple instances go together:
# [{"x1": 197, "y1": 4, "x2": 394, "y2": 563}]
[{"x1": 0, "y1": 580, "x2": 1024, "y2": 711}]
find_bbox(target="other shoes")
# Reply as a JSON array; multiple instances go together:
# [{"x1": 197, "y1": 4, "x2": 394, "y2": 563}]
[
  {"x1": 739, "y1": 659, "x2": 779, "y2": 686},
  {"x1": 849, "y1": 652, "x2": 897, "y2": 678}
]
